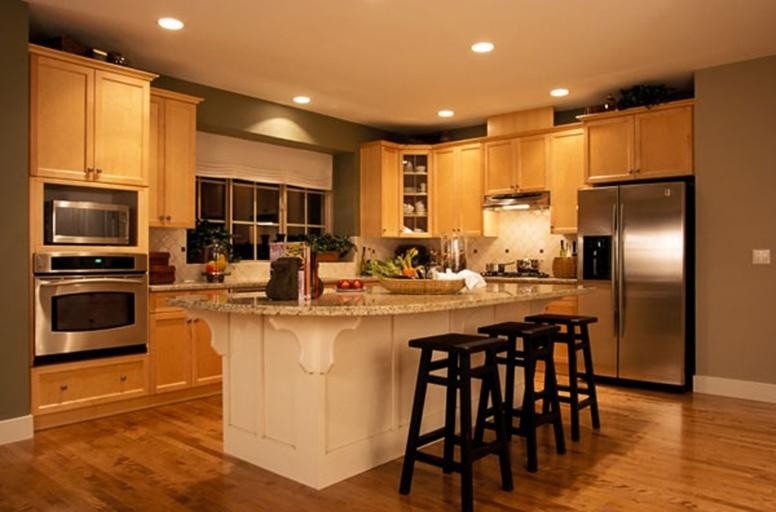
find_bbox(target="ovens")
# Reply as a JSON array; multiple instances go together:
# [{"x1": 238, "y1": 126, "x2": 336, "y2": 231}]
[{"x1": 30, "y1": 251, "x2": 148, "y2": 368}]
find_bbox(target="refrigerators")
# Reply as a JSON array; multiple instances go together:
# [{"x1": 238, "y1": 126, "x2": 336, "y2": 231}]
[{"x1": 576, "y1": 182, "x2": 691, "y2": 396}]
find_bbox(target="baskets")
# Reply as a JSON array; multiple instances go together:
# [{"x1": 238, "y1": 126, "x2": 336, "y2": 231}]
[{"x1": 380, "y1": 277, "x2": 465, "y2": 294}]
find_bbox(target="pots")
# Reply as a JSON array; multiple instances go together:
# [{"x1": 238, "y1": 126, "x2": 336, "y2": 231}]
[
  {"x1": 517, "y1": 259, "x2": 542, "y2": 271},
  {"x1": 485, "y1": 261, "x2": 515, "y2": 272}
]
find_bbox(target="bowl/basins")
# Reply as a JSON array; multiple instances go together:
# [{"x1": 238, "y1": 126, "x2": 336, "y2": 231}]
[
  {"x1": 416, "y1": 166, "x2": 425, "y2": 172},
  {"x1": 414, "y1": 200, "x2": 424, "y2": 215}
]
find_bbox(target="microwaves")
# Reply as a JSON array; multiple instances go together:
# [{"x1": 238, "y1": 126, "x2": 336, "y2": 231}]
[{"x1": 43, "y1": 199, "x2": 130, "y2": 245}]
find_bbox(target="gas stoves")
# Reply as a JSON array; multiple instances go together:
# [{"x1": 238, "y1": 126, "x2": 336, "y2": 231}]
[{"x1": 481, "y1": 270, "x2": 549, "y2": 278}]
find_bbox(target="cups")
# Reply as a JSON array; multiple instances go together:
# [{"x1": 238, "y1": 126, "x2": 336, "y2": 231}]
[
  {"x1": 584, "y1": 106, "x2": 592, "y2": 115},
  {"x1": 440, "y1": 233, "x2": 467, "y2": 274},
  {"x1": 420, "y1": 182, "x2": 426, "y2": 192}
]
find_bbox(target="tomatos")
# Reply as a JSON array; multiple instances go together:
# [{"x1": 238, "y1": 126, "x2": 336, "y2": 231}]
[{"x1": 336, "y1": 279, "x2": 364, "y2": 291}]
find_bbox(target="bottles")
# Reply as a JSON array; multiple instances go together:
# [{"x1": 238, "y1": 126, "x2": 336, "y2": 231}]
[
  {"x1": 360, "y1": 247, "x2": 377, "y2": 276},
  {"x1": 601, "y1": 94, "x2": 617, "y2": 112},
  {"x1": 560, "y1": 240, "x2": 577, "y2": 256}
]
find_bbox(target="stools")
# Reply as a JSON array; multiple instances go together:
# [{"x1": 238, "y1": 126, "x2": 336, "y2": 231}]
[
  {"x1": 521, "y1": 311, "x2": 603, "y2": 443},
  {"x1": 471, "y1": 321, "x2": 567, "y2": 476},
  {"x1": 394, "y1": 330, "x2": 517, "y2": 511}
]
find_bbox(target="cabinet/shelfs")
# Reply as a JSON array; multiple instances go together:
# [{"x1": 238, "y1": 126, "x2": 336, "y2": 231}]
[
  {"x1": 548, "y1": 129, "x2": 597, "y2": 234},
  {"x1": 31, "y1": 351, "x2": 153, "y2": 431},
  {"x1": 149, "y1": 288, "x2": 228, "y2": 407},
  {"x1": 28, "y1": 42, "x2": 161, "y2": 187},
  {"x1": 359, "y1": 138, "x2": 430, "y2": 239},
  {"x1": 485, "y1": 136, "x2": 550, "y2": 195},
  {"x1": 431, "y1": 139, "x2": 497, "y2": 238},
  {"x1": 147, "y1": 91, "x2": 202, "y2": 231},
  {"x1": 575, "y1": 98, "x2": 694, "y2": 183}
]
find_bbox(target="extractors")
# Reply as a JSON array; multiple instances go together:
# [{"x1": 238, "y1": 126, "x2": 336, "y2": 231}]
[{"x1": 481, "y1": 192, "x2": 550, "y2": 210}]
